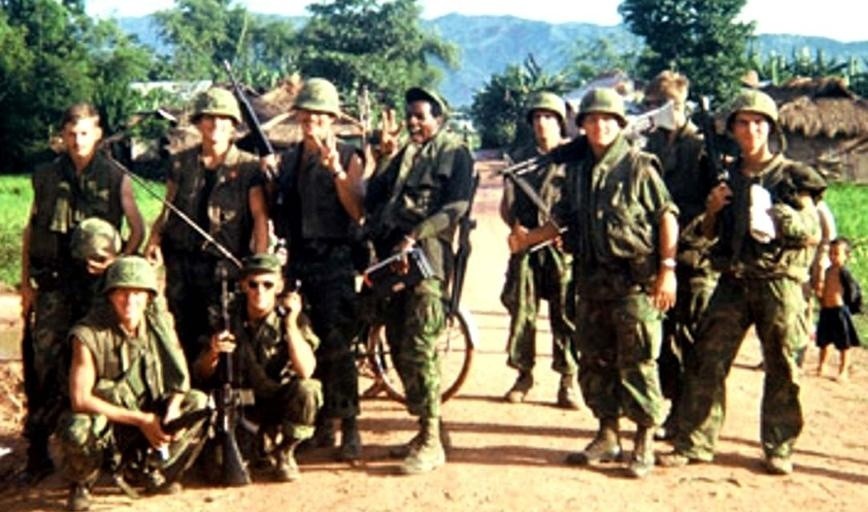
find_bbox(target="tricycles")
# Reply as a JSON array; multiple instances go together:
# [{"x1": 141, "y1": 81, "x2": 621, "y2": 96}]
[{"x1": 290, "y1": 167, "x2": 482, "y2": 404}]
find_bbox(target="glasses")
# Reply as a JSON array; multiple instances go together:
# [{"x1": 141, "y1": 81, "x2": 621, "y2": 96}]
[{"x1": 248, "y1": 279, "x2": 275, "y2": 289}]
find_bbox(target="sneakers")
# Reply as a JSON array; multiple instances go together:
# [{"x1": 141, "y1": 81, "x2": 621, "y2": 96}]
[
  {"x1": 506, "y1": 375, "x2": 534, "y2": 402},
  {"x1": 391, "y1": 439, "x2": 447, "y2": 474},
  {"x1": 558, "y1": 387, "x2": 579, "y2": 409},
  {"x1": 769, "y1": 453, "x2": 793, "y2": 476},
  {"x1": 67, "y1": 480, "x2": 91, "y2": 512},
  {"x1": 567, "y1": 442, "x2": 690, "y2": 478},
  {"x1": 275, "y1": 447, "x2": 299, "y2": 478},
  {"x1": 337, "y1": 435, "x2": 360, "y2": 460}
]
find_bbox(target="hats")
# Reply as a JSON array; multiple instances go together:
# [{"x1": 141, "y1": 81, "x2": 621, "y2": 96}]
[
  {"x1": 244, "y1": 254, "x2": 281, "y2": 276},
  {"x1": 404, "y1": 87, "x2": 450, "y2": 120}
]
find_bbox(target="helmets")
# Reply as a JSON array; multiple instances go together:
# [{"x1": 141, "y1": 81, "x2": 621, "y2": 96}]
[
  {"x1": 526, "y1": 91, "x2": 566, "y2": 130},
  {"x1": 291, "y1": 77, "x2": 342, "y2": 119},
  {"x1": 574, "y1": 87, "x2": 627, "y2": 128},
  {"x1": 106, "y1": 256, "x2": 156, "y2": 293},
  {"x1": 725, "y1": 90, "x2": 778, "y2": 133},
  {"x1": 188, "y1": 88, "x2": 244, "y2": 126},
  {"x1": 72, "y1": 217, "x2": 124, "y2": 259}
]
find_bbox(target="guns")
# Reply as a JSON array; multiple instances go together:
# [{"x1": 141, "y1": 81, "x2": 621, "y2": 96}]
[
  {"x1": 90, "y1": 404, "x2": 234, "y2": 468},
  {"x1": 692, "y1": 114, "x2": 745, "y2": 288},
  {"x1": 222, "y1": 60, "x2": 287, "y2": 213},
  {"x1": 493, "y1": 104, "x2": 675, "y2": 179},
  {"x1": 208, "y1": 266, "x2": 249, "y2": 485}
]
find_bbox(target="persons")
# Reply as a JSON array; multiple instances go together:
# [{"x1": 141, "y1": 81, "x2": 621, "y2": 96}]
[
  {"x1": 16, "y1": 75, "x2": 475, "y2": 510},
  {"x1": 498, "y1": 69, "x2": 834, "y2": 477},
  {"x1": 816, "y1": 235, "x2": 864, "y2": 383}
]
